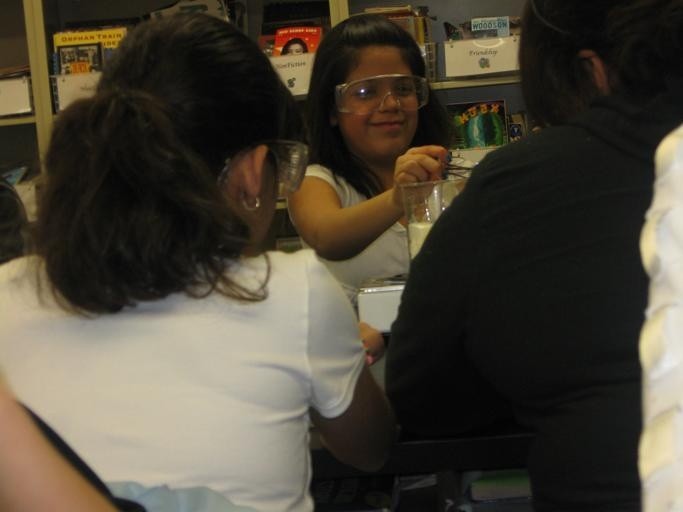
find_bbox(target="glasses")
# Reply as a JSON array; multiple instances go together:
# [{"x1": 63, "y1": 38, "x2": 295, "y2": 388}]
[
  {"x1": 335, "y1": 73, "x2": 427, "y2": 115},
  {"x1": 218, "y1": 139, "x2": 308, "y2": 192}
]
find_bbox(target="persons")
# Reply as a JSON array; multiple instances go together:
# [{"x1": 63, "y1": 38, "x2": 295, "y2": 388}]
[
  {"x1": 0, "y1": 6, "x2": 400, "y2": 511},
  {"x1": 282, "y1": 10, "x2": 458, "y2": 293},
  {"x1": 382, "y1": 0, "x2": 683, "y2": 511},
  {"x1": 279, "y1": 38, "x2": 309, "y2": 57}
]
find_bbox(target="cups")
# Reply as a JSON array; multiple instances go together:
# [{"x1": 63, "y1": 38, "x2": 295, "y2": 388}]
[{"x1": 401, "y1": 177, "x2": 460, "y2": 270}]
